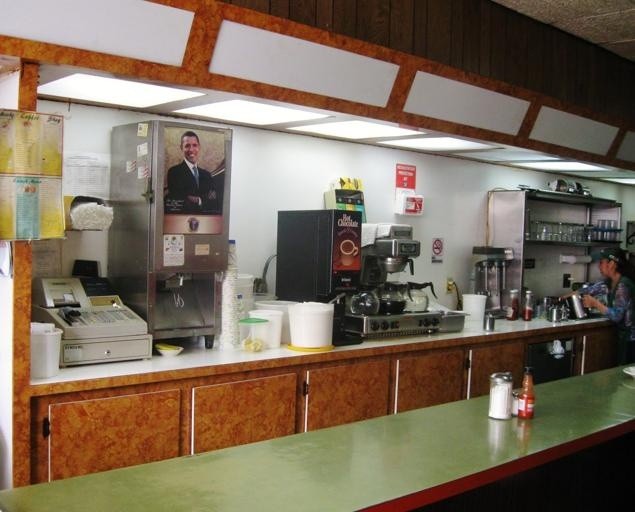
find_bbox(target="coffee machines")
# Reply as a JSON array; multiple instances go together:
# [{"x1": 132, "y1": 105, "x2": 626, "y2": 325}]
[
  {"x1": 275, "y1": 206, "x2": 365, "y2": 345},
  {"x1": 345, "y1": 221, "x2": 443, "y2": 336}
]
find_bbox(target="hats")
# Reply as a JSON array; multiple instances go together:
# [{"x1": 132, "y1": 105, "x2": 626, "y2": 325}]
[{"x1": 590, "y1": 246, "x2": 620, "y2": 263}]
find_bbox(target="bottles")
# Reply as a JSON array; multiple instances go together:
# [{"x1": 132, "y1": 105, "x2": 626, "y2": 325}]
[
  {"x1": 520, "y1": 366, "x2": 536, "y2": 419},
  {"x1": 512, "y1": 393, "x2": 519, "y2": 418},
  {"x1": 506, "y1": 289, "x2": 520, "y2": 321},
  {"x1": 445, "y1": 278, "x2": 458, "y2": 311},
  {"x1": 488, "y1": 370, "x2": 511, "y2": 420},
  {"x1": 523, "y1": 290, "x2": 534, "y2": 321}
]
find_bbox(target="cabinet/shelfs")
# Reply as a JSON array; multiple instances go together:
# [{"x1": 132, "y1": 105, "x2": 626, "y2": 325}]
[{"x1": 13, "y1": 321, "x2": 621, "y2": 487}]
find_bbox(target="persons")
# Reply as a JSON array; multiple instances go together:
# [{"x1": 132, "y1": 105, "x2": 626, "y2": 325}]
[
  {"x1": 558, "y1": 248, "x2": 635, "y2": 366},
  {"x1": 167, "y1": 131, "x2": 218, "y2": 213}
]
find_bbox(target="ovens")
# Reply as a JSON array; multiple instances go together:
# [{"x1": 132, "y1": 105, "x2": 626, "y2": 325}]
[{"x1": 525, "y1": 337, "x2": 575, "y2": 383}]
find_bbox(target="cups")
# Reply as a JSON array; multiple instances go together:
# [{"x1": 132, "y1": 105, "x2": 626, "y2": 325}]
[
  {"x1": 340, "y1": 239, "x2": 359, "y2": 267},
  {"x1": 221, "y1": 239, "x2": 242, "y2": 346},
  {"x1": 572, "y1": 294, "x2": 591, "y2": 319},
  {"x1": 462, "y1": 293, "x2": 487, "y2": 331},
  {"x1": 536, "y1": 295, "x2": 569, "y2": 323},
  {"x1": 532, "y1": 219, "x2": 618, "y2": 242}
]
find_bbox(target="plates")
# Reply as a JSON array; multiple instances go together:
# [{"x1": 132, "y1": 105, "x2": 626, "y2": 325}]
[
  {"x1": 155, "y1": 346, "x2": 184, "y2": 357},
  {"x1": 622, "y1": 365, "x2": 635, "y2": 379}
]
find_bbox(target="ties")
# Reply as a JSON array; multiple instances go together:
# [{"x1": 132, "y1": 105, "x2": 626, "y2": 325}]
[{"x1": 191, "y1": 165, "x2": 200, "y2": 188}]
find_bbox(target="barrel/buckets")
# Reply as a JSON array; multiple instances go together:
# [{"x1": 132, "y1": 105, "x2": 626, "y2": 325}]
[
  {"x1": 463, "y1": 293, "x2": 488, "y2": 324},
  {"x1": 31, "y1": 329, "x2": 63, "y2": 378}
]
